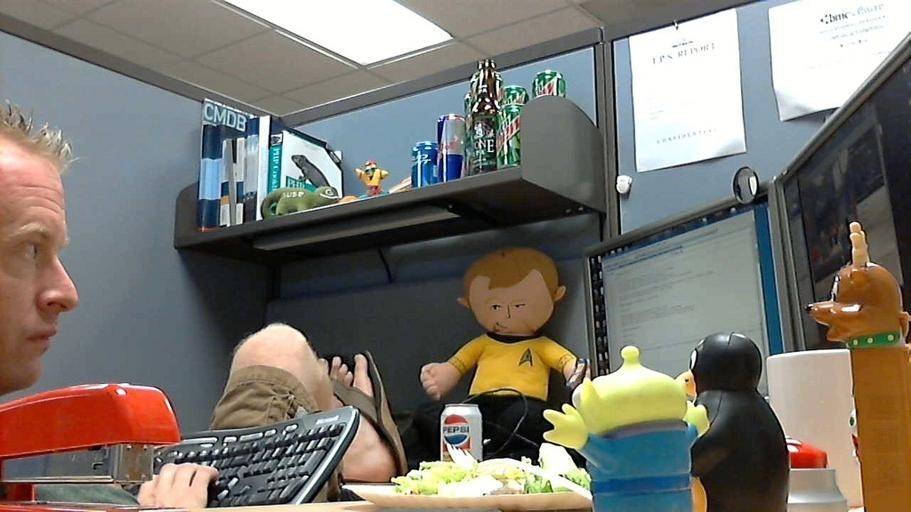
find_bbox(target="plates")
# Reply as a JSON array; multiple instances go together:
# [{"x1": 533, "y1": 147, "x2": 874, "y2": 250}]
[{"x1": 342, "y1": 484, "x2": 592, "y2": 511}]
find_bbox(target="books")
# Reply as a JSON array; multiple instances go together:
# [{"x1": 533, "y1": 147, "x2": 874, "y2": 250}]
[{"x1": 198, "y1": 101, "x2": 341, "y2": 232}]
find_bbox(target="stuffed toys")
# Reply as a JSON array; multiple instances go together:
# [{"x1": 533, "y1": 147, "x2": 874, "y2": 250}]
[{"x1": 420, "y1": 249, "x2": 591, "y2": 466}]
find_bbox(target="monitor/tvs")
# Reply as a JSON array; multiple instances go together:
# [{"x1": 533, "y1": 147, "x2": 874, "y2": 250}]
[
  {"x1": 775, "y1": 31, "x2": 910, "y2": 349},
  {"x1": 583, "y1": 178, "x2": 795, "y2": 404}
]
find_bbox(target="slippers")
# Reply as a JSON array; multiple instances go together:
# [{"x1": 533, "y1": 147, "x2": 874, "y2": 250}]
[{"x1": 331, "y1": 351, "x2": 408, "y2": 477}]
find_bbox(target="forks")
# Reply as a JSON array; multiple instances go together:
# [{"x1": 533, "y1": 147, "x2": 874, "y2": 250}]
[{"x1": 446, "y1": 443, "x2": 593, "y2": 500}]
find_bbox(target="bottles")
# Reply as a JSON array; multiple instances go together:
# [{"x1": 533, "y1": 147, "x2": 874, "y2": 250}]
[
  {"x1": 468, "y1": 57, "x2": 499, "y2": 170},
  {"x1": 500, "y1": 104, "x2": 521, "y2": 167},
  {"x1": 438, "y1": 404, "x2": 483, "y2": 465},
  {"x1": 410, "y1": 144, "x2": 437, "y2": 188},
  {"x1": 503, "y1": 87, "x2": 527, "y2": 103},
  {"x1": 534, "y1": 72, "x2": 565, "y2": 99},
  {"x1": 431, "y1": 114, "x2": 465, "y2": 182}
]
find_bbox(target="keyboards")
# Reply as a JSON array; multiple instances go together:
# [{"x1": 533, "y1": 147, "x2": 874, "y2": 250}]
[{"x1": 118, "y1": 406, "x2": 361, "y2": 506}]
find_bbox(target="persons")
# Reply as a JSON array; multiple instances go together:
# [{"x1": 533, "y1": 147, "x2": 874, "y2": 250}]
[{"x1": 0, "y1": 99, "x2": 407, "y2": 509}]
